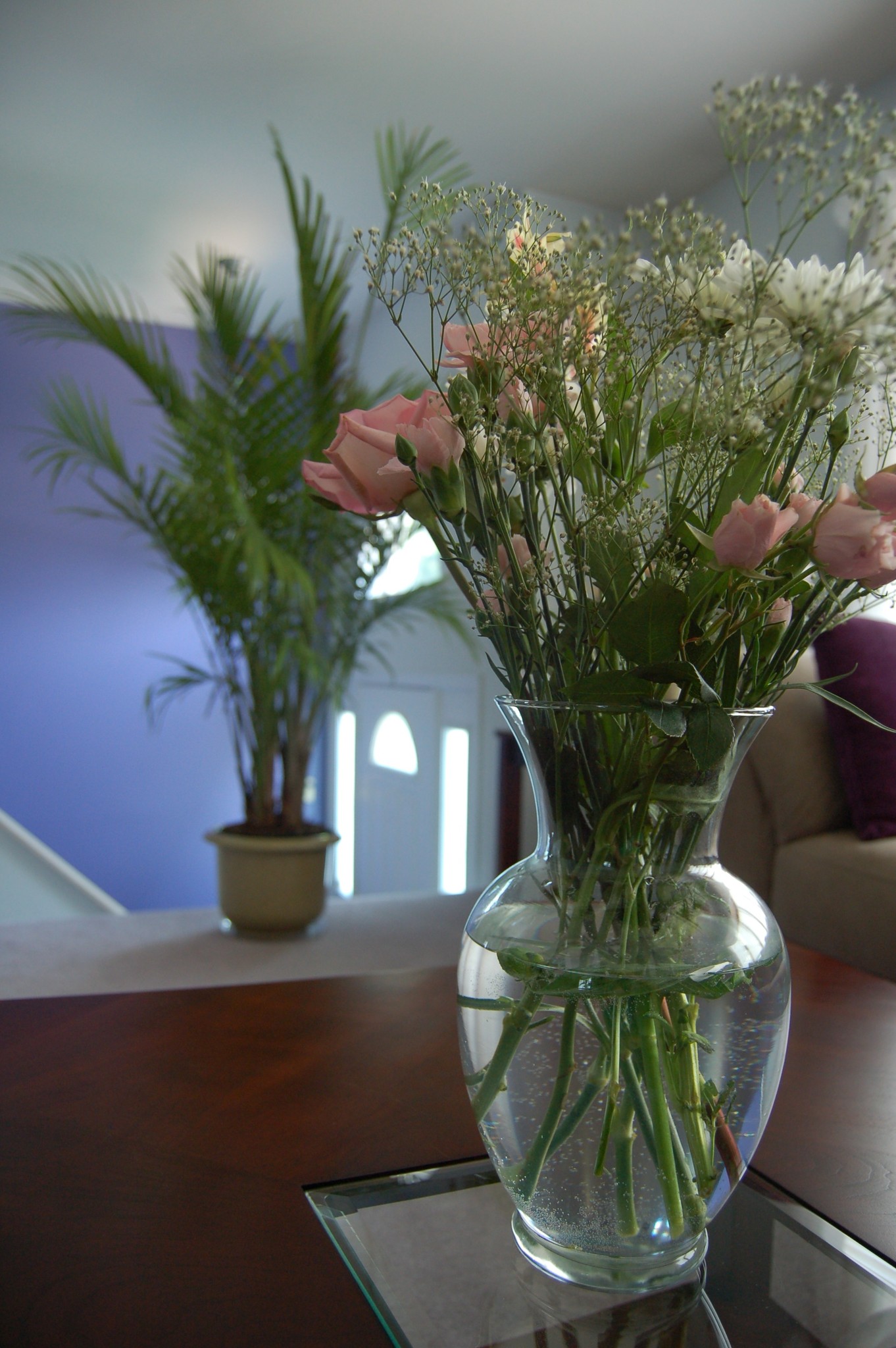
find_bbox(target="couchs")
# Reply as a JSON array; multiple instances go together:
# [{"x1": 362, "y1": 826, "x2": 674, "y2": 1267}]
[{"x1": 718, "y1": 650, "x2": 896, "y2": 982}]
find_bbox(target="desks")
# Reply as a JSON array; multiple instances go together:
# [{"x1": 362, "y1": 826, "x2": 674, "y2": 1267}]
[{"x1": 303, "y1": 1153, "x2": 896, "y2": 1347}]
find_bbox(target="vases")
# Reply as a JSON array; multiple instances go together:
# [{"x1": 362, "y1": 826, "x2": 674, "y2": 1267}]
[{"x1": 456, "y1": 695, "x2": 791, "y2": 1291}]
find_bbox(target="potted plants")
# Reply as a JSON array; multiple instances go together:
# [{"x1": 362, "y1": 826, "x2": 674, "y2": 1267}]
[{"x1": 1, "y1": 113, "x2": 476, "y2": 936}]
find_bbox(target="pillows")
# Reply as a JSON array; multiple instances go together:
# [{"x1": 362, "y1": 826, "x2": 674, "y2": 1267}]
[{"x1": 816, "y1": 619, "x2": 896, "y2": 839}]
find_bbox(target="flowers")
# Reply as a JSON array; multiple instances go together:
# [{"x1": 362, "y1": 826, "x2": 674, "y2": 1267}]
[{"x1": 302, "y1": 70, "x2": 896, "y2": 1243}]
